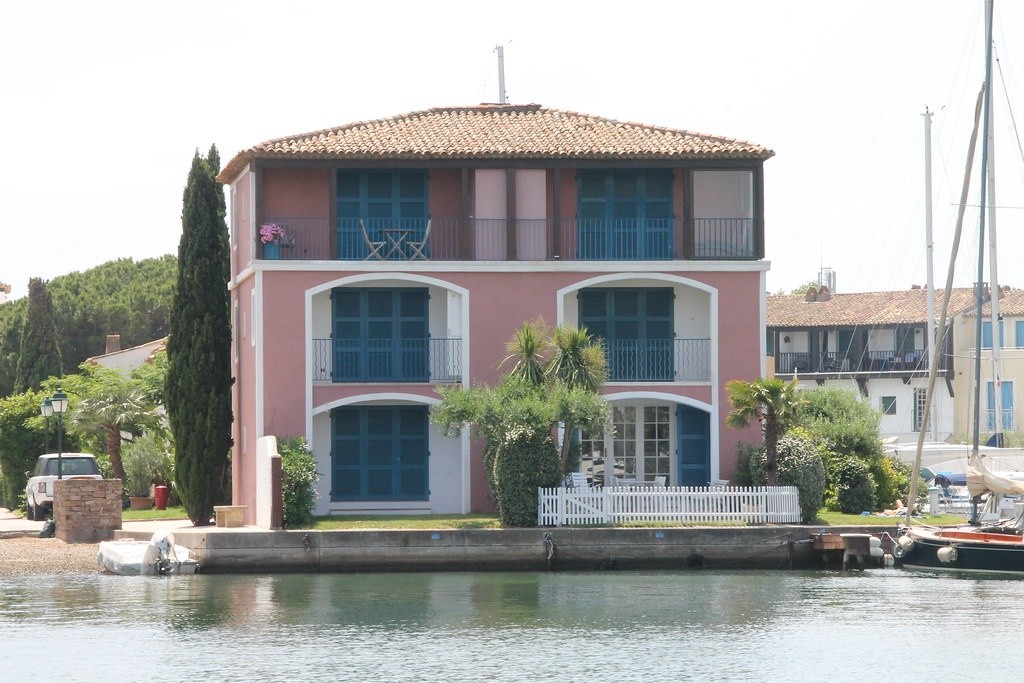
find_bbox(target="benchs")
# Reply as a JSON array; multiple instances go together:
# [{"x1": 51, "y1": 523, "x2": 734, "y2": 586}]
[
  {"x1": 692, "y1": 479, "x2": 729, "y2": 504},
  {"x1": 214, "y1": 505, "x2": 249, "y2": 528}
]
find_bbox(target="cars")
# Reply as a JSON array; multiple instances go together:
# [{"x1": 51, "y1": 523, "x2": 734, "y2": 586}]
[{"x1": 24, "y1": 453, "x2": 103, "y2": 521}]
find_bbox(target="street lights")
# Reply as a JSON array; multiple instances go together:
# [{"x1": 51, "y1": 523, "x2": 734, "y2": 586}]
[
  {"x1": 51, "y1": 388, "x2": 69, "y2": 479},
  {"x1": 40, "y1": 396, "x2": 55, "y2": 454}
]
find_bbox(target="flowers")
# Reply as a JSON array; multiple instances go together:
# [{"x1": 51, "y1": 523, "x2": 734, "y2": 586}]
[{"x1": 260, "y1": 224, "x2": 286, "y2": 244}]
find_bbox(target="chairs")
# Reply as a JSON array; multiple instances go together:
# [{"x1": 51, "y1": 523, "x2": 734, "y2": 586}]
[
  {"x1": 889, "y1": 351, "x2": 915, "y2": 369},
  {"x1": 822, "y1": 358, "x2": 840, "y2": 371},
  {"x1": 570, "y1": 473, "x2": 604, "y2": 506},
  {"x1": 406, "y1": 220, "x2": 432, "y2": 260},
  {"x1": 359, "y1": 219, "x2": 387, "y2": 261},
  {"x1": 794, "y1": 355, "x2": 807, "y2": 372},
  {"x1": 608, "y1": 475, "x2": 666, "y2": 507}
]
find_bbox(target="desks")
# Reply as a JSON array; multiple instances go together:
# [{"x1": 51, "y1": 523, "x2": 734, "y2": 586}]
[
  {"x1": 619, "y1": 481, "x2": 660, "y2": 493},
  {"x1": 379, "y1": 229, "x2": 413, "y2": 259}
]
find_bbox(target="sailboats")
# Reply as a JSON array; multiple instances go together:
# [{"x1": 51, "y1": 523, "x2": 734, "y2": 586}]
[{"x1": 894, "y1": 0, "x2": 1024, "y2": 573}]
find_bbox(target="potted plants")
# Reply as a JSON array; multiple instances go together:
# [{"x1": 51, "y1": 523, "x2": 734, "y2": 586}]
[{"x1": 122, "y1": 436, "x2": 160, "y2": 511}]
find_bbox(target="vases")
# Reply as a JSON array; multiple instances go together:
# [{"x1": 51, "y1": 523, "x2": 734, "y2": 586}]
[{"x1": 263, "y1": 237, "x2": 280, "y2": 260}]
